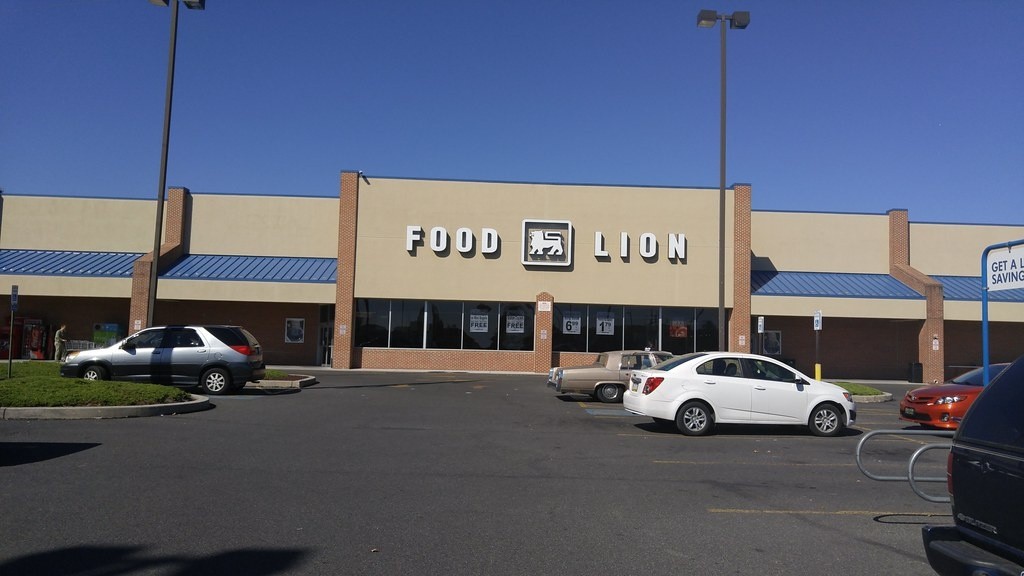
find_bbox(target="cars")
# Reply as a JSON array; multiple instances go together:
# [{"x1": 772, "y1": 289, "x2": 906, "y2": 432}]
[
  {"x1": 899, "y1": 362, "x2": 1013, "y2": 431},
  {"x1": 622, "y1": 350, "x2": 857, "y2": 438},
  {"x1": 544, "y1": 348, "x2": 676, "y2": 404}
]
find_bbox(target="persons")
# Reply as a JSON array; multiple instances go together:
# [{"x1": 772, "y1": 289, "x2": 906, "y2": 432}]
[
  {"x1": 54, "y1": 324, "x2": 67, "y2": 361},
  {"x1": 725, "y1": 363, "x2": 737, "y2": 376}
]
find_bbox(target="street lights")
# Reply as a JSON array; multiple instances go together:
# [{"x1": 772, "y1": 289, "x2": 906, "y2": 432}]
[
  {"x1": 695, "y1": 8, "x2": 757, "y2": 351},
  {"x1": 141, "y1": 0, "x2": 208, "y2": 329}
]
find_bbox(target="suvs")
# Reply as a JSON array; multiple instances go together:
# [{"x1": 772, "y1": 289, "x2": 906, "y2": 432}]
[
  {"x1": 921, "y1": 351, "x2": 1024, "y2": 576},
  {"x1": 58, "y1": 323, "x2": 268, "y2": 396}
]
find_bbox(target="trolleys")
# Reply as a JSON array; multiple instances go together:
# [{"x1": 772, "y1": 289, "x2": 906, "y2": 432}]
[{"x1": 60, "y1": 339, "x2": 105, "y2": 363}]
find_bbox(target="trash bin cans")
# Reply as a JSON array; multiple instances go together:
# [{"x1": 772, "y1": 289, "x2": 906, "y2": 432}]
[
  {"x1": 909, "y1": 363, "x2": 923, "y2": 383},
  {"x1": 782, "y1": 359, "x2": 796, "y2": 378}
]
